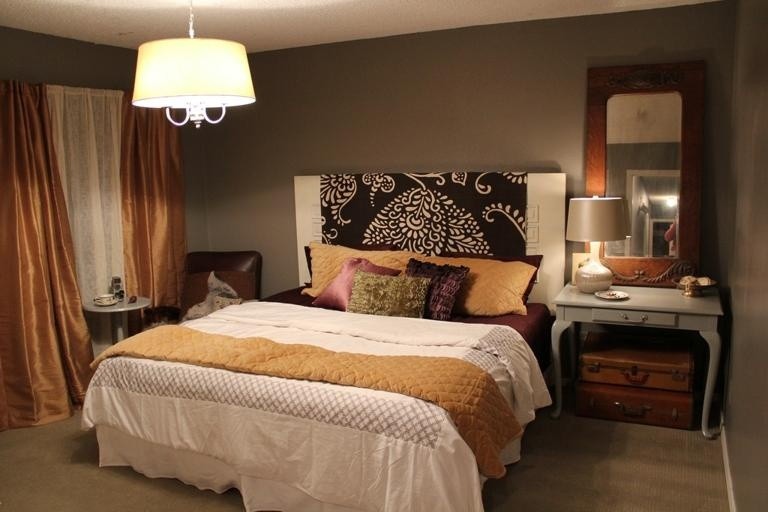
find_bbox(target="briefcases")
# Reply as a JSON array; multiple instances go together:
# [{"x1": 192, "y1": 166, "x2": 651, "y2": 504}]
[
  {"x1": 578, "y1": 331, "x2": 694, "y2": 394},
  {"x1": 574, "y1": 379, "x2": 693, "y2": 430}
]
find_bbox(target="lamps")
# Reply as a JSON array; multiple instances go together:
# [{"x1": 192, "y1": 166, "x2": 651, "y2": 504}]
[
  {"x1": 132, "y1": 1, "x2": 257, "y2": 129},
  {"x1": 566, "y1": 195, "x2": 626, "y2": 294}
]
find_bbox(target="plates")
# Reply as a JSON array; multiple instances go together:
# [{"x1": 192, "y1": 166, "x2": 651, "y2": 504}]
[
  {"x1": 595, "y1": 290, "x2": 628, "y2": 300},
  {"x1": 95, "y1": 302, "x2": 117, "y2": 306}
]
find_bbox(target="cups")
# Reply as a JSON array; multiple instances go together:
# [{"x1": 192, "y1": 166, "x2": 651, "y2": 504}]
[{"x1": 96, "y1": 293, "x2": 116, "y2": 303}]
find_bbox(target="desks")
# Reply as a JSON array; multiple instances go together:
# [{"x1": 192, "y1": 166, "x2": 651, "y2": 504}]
[
  {"x1": 550, "y1": 281, "x2": 724, "y2": 439},
  {"x1": 83, "y1": 296, "x2": 151, "y2": 342}
]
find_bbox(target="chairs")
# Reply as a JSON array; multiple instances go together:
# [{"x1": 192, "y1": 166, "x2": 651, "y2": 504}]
[{"x1": 145, "y1": 250, "x2": 261, "y2": 326}]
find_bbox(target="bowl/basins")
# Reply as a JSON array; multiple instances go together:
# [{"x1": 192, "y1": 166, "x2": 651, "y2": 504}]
[{"x1": 670, "y1": 275, "x2": 717, "y2": 292}]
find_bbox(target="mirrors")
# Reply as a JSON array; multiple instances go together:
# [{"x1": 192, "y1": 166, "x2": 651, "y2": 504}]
[{"x1": 585, "y1": 61, "x2": 705, "y2": 289}]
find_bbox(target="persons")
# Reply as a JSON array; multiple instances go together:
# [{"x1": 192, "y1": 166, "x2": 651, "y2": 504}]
[{"x1": 664, "y1": 222, "x2": 677, "y2": 251}]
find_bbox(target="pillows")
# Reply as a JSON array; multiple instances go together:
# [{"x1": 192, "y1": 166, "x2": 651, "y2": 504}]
[{"x1": 300, "y1": 243, "x2": 543, "y2": 321}]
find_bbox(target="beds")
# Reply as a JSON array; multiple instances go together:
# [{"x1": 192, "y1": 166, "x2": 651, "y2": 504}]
[{"x1": 80, "y1": 170, "x2": 568, "y2": 512}]
